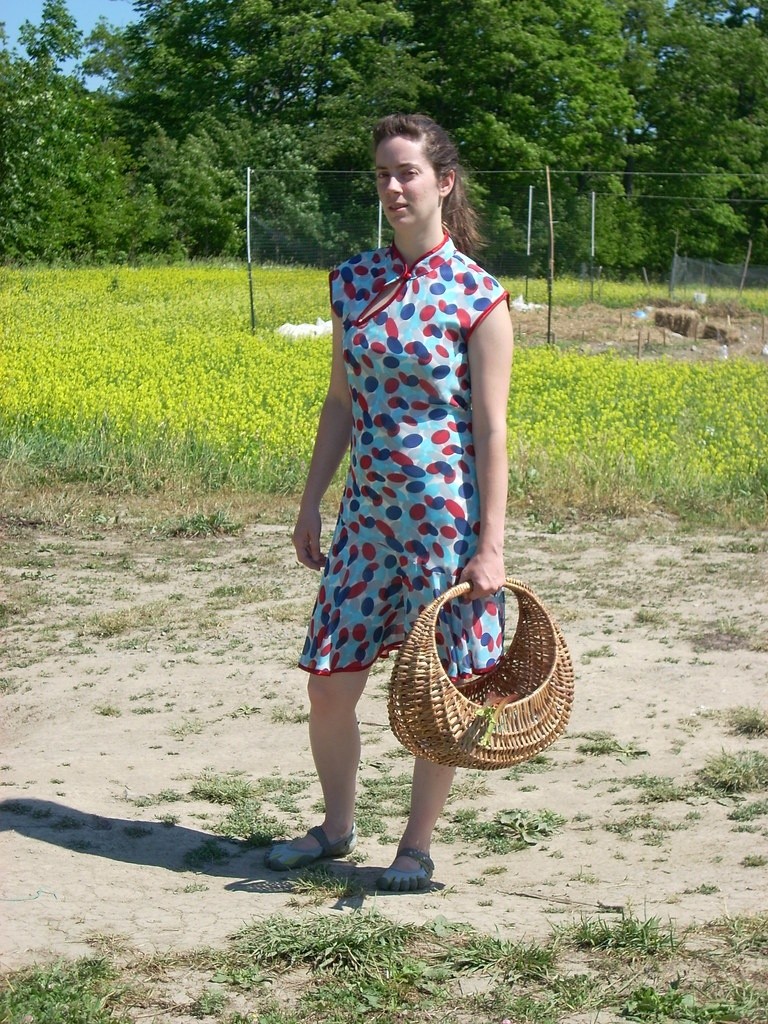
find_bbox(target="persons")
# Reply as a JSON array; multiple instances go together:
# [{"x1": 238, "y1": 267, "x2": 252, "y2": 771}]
[{"x1": 265, "y1": 114, "x2": 514, "y2": 891}]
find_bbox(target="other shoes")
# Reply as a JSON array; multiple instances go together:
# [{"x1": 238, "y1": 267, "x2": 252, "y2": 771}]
[
  {"x1": 264, "y1": 822, "x2": 358, "y2": 871},
  {"x1": 380, "y1": 849, "x2": 434, "y2": 892}
]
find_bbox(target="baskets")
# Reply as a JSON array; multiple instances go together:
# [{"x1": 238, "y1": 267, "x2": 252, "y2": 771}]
[{"x1": 388, "y1": 578, "x2": 573, "y2": 770}]
